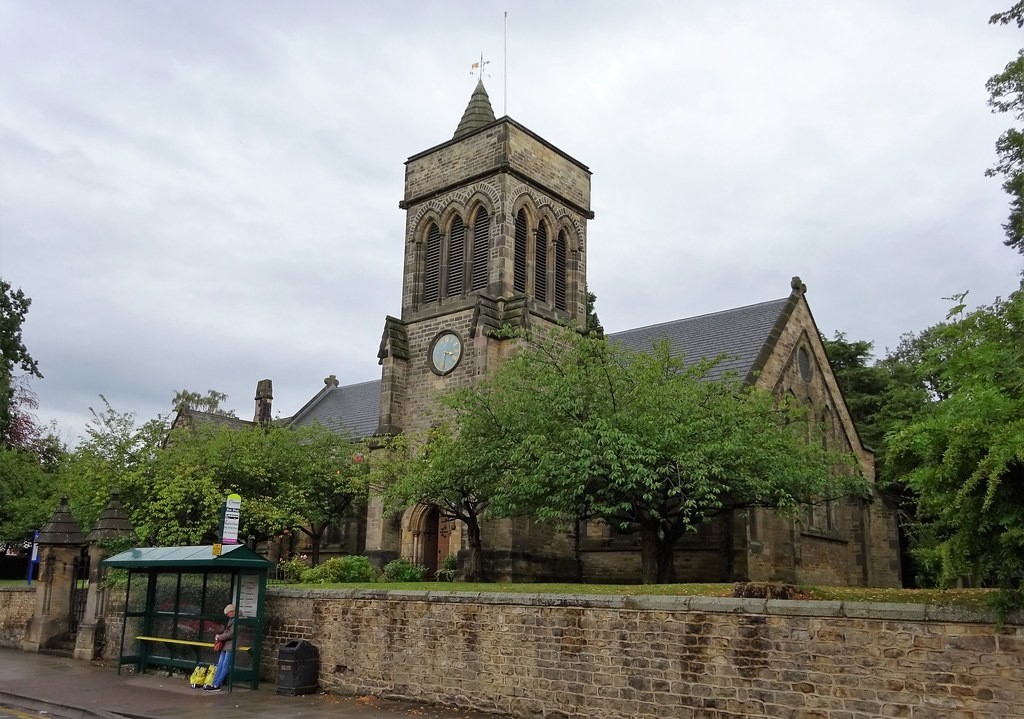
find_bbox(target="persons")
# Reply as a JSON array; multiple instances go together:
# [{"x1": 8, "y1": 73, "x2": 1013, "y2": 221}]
[{"x1": 206, "y1": 604, "x2": 247, "y2": 692}]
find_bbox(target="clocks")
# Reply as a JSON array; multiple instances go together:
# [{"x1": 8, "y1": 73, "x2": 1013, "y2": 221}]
[{"x1": 427, "y1": 329, "x2": 464, "y2": 376}]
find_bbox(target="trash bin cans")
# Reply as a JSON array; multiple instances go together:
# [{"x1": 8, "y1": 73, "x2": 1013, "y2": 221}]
[{"x1": 276, "y1": 639, "x2": 321, "y2": 697}]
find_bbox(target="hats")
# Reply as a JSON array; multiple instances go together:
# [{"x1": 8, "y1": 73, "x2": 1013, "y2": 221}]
[{"x1": 223, "y1": 604, "x2": 236, "y2": 615}]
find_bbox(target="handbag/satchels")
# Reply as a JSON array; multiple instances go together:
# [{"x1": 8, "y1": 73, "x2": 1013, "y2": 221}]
[
  {"x1": 191, "y1": 662, "x2": 207, "y2": 688},
  {"x1": 203, "y1": 663, "x2": 217, "y2": 690},
  {"x1": 214, "y1": 641, "x2": 223, "y2": 651}
]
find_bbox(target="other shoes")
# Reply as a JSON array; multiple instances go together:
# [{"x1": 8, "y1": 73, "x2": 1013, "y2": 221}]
[{"x1": 206, "y1": 685, "x2": 221, "y2": 692}]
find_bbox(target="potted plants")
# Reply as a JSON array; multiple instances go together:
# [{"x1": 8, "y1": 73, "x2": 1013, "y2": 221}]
[{"x1": 434, "y1": 569, "x2": 455, "y2": 582}]
